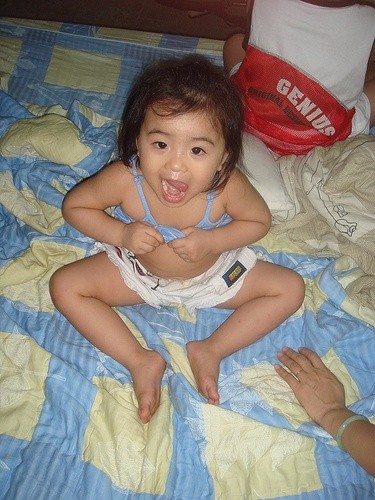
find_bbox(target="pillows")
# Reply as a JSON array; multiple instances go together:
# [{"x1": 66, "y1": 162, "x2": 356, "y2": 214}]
[{"x1": 235, "y1": 133, "x2": 296, "y2": 211}]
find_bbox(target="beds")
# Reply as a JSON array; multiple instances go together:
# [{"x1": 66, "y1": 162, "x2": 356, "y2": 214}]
[{"x1": 0, "y1": 18, "x2": 375, "y2": 500}]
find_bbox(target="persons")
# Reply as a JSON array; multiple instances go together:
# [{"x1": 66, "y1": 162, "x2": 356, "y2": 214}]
[
  {"x1": 223, "y1": 0, "x2": 375, "y2": 156},
  {"x1": 49, "y1": 58, "x2": 304, "y2": 422},
  {"x1": 275, "y1": 346, "x2": 375, "y2": 480}
]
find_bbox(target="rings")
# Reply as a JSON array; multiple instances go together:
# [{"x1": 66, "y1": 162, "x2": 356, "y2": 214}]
[{"x1": 295, "y1": 368, "x2": 303, "y2": 377}]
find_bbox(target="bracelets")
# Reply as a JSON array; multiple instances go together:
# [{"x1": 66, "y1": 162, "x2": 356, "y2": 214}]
[{"x1": 336, "y1": 413, "x2": 371, "y2": 453}]
